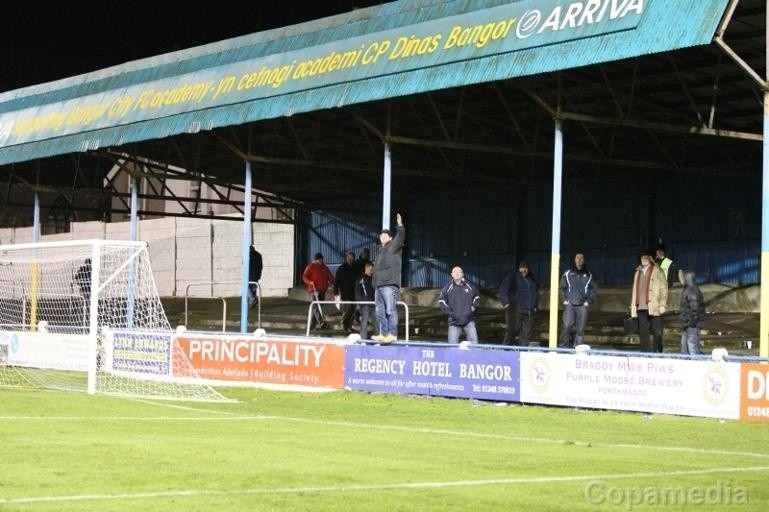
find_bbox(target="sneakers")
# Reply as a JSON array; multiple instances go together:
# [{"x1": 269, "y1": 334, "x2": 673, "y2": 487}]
[
  {"x1": 370, "y1": 334, "x2": 386, "y2": 341},
  {"x1": 379, "y1": 334, "x2": 398, "y2": 343}
]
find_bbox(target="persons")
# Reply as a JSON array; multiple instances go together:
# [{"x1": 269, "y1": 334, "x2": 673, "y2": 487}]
[
  {"x1": 629, "y1": 254, "x2": 669, "y2": 353},
  {"x1": 498, "y1": 259, "x2": 539, "y2": 346},
  {"x1": 437, "y1": 265, "x2": 481, "y2": 344},
  {"x1": 249, "y1": 245, "x2": 263, "y2": 309},
  {"x1": 370, "y1": 213, "x2": 406, "y2": 344},
  {"x1": 679, "y1": 270, "x2": 705, "y2": 354},
  {"x1": 653, "y1": 245, "x2": 676, "y2": 289},
  {"x1": 75, "y1": 258, "x2": 92, "y2": 321},
  {"x1": 302, "y1": 252, "x2": 335, "y2": 330},
  {"x1": 333, "y1": 251, "x2": 360, "y2": 333},
  {"x1": 355, "y1": 248, "x2": 372, "y2": 272},
  {"x1": 354, "y1": 262, "x2": 377, "y2": 340},
  {"x1": 558, "y1": 252, "x2": 598, "y2": 349}
]
